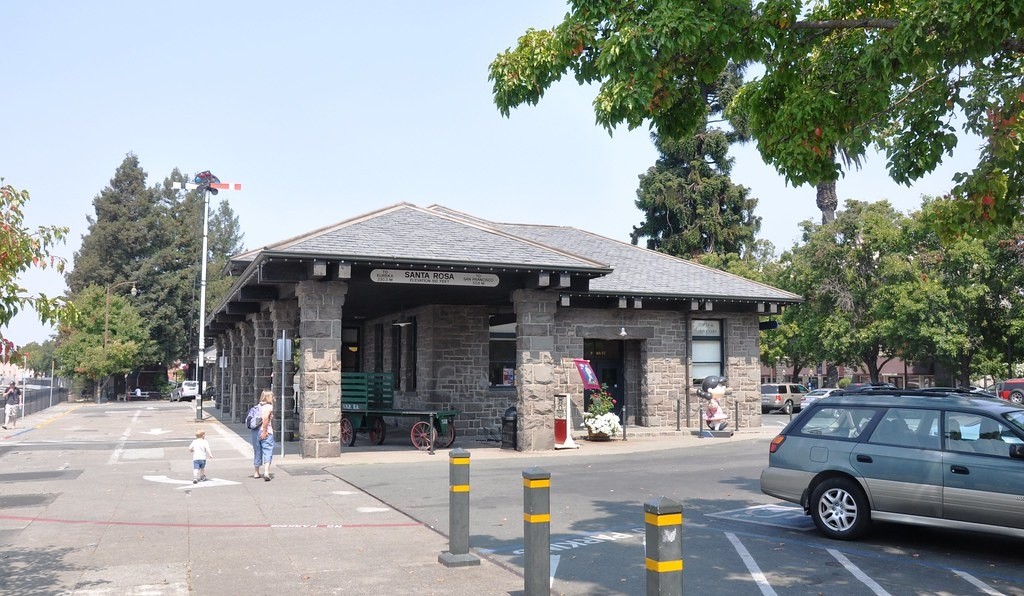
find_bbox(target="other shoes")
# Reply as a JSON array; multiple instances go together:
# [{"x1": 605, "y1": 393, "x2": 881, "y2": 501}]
[
  {"x1": 254, "y1": 474, "x2": 259, "y2": 478},
  {"x1": 193, "y1": 477, "x2": 198, "y2": 484},
  {"x1": 200, "y1": 475, "x2": 206, "y2": 481},
  {"x1": 263, "y1": 476, "x2": 271, "y2": 482},
  {"x1": 2, "y1": 426, "x2": 7, "y2": 430}
]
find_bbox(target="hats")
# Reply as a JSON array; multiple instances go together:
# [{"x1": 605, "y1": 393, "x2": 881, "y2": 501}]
[{"x1": 10, "y1": 381, "x2": 16, "y2": 384}]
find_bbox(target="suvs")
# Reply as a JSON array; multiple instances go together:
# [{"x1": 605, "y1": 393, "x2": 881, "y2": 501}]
[{"x1": 759, "y1": 386, "x2": 1024, "y2": 544}]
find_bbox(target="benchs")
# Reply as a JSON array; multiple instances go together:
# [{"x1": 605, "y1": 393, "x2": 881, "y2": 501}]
[
  {"x1": 127, "y1": 391, "x2": 150, "y2": 401},
  {"x1": 861, "y1": 419, "x2": 920, "y2": 448}
]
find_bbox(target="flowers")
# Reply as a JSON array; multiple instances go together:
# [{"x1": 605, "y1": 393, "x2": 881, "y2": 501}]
[{"x1": 588, "y1": 384, "x2": 616, "y2": 415}]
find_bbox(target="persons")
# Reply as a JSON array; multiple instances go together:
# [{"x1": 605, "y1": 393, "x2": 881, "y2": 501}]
[
  {"x1": 254, "y1": 390, "x2": 274, "y2": 481},
  {"x1": 696, "y1": 376, "x2": 728, "y2": 431},
  {"x1": 125, "y1": 385, "x2": 131, "y2": 401},
  {"x1": 81, "y1": 386, "x2": 89, "y2": 401},
  {"x1": 585, "y1": 365, "x2": 596, "y2": 383},
  {"x1": 189, "y1": 428, "x2": 213, "y2": 484},
  {"x1": 2, "y1": 381, "x2": 21, "y2": 430},
  {"x1": 135, "y1": 387, "x2": 141, "y2": 396}
]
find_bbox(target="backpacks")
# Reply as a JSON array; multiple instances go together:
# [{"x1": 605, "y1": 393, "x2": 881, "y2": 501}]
[{"x1": 244, "y1": 403, "x2": 271, "y2": 430}]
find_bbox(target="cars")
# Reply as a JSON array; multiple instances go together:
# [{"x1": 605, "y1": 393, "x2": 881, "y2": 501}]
[
  {"x1": 799, "y1": 388, "x2": 845, "y2": 412},
  {"x1": 846, "y1": 382, "x2": 873, "y2": 395},
  {"x1": 970, "y1": 377, "x2": 1024, "y2": 405},
  {"x1": 761, "y1": 382, "x2": 810, "y2": 415},
  {"x1": 171, "y1": 380, "x2": 199, "y2": 402}
]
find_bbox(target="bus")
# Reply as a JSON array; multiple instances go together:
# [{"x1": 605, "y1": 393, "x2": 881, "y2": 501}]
[{"x1": 21, "y1": 377, "x2": 61, "y2": 390}]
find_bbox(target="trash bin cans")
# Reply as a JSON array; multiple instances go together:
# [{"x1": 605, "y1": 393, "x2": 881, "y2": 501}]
[{"x1": 500, "y1": 406, "x2": 517, "y2": 450}]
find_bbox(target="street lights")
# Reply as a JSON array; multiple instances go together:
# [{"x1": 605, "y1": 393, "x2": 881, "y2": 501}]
[{"x1": 92, "y1": 278, "x2": 138, "y2": 401}]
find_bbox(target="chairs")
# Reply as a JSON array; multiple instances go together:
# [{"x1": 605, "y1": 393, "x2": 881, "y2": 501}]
[
  {"x1": 973, "y1": 417, "x2": 1009, "y2": 457},
  {"x1": 947, "y1": 419, "x2": 974, "y2": 453}
]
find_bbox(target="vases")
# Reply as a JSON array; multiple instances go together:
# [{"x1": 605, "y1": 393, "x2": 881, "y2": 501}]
[{"x1": 587, "y1": 419, "x2": 611, "y2": 441}]
